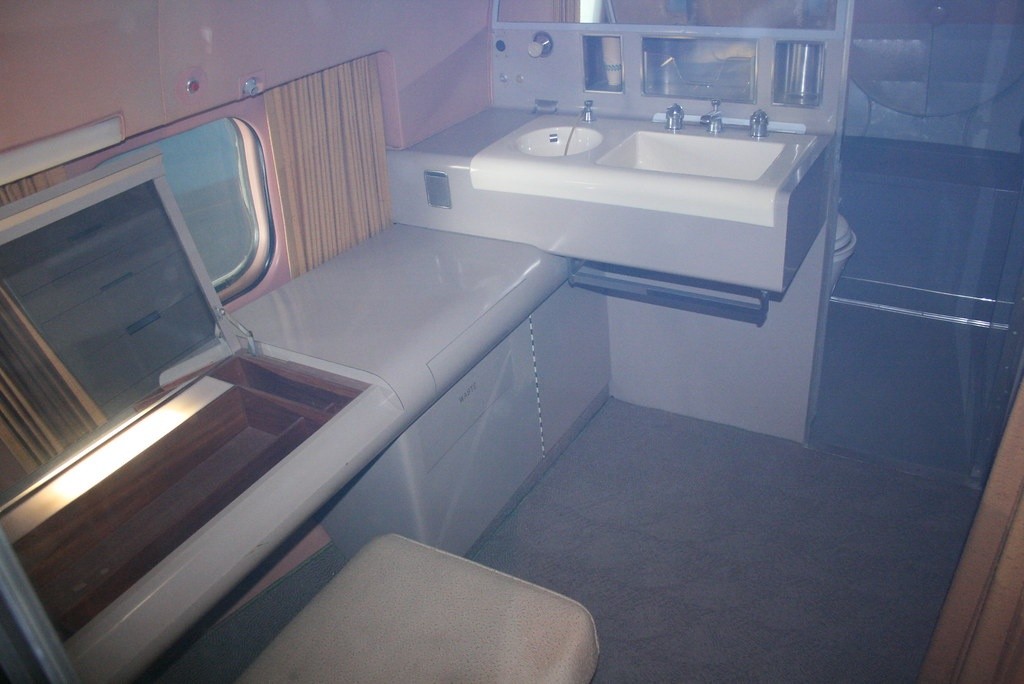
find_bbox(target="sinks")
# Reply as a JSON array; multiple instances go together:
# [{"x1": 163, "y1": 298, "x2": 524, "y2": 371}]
[
  {"x1": 594, "y1": 130, "x2": 786, "y2": 182},
  {"x1": 515, "y1": 126, "x2": 603, "y2": 157}
]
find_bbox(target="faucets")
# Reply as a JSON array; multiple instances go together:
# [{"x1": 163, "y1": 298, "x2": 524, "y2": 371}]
[
  {"x1": 699, "y1": 99, "x2": 724, "y2": 133},
  {"x1": 579, "y1": 100, "x2": 595, "y2": 123}
]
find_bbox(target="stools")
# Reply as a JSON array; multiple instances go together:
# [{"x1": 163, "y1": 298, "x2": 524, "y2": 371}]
[{"x1": 232, "y1": 531, "x2": 599, "y2": 684}]
